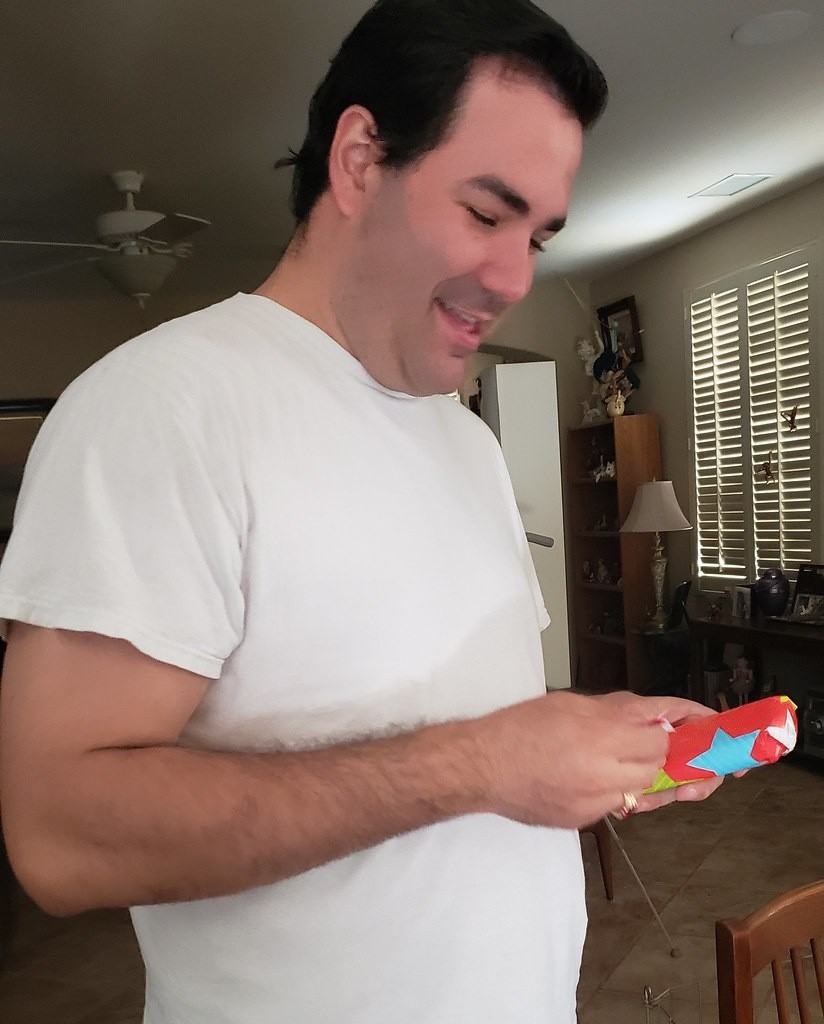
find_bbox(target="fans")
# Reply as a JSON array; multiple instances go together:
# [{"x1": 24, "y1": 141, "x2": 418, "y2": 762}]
[{"x1": 0, "y1": 170, "x2": 210, "y2": 288}]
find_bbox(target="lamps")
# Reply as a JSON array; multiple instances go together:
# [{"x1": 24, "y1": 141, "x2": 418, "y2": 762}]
[
  {"x1": 95, "y1": 253, "x2": 173, "y2": 309},
  {"x1": 619, "y1": 476, "x2": 693, "y2": 632}
]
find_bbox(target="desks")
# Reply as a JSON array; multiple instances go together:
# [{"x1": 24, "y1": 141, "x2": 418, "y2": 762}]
[{"x1": 690, "y1": 613, "x2": 824, "y2": 775}]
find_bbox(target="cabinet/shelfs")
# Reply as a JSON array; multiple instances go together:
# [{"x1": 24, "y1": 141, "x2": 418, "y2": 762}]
[
  {"x1": 478, "y1": 359, "x2": 570, "y2": 690},
  {"x1": 565, "y1": 414, "x2": 664, "y2": 693}
]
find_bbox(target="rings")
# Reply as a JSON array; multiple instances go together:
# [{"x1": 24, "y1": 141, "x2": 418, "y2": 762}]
[{"x1": 611, "y1": 793, "x2": 637, "y2": 821}]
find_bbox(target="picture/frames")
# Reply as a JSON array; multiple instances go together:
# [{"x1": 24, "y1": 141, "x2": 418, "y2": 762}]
[
  {"x1": 596, "y1": 294, "x2": 643, "y2": 365},
  {"x1": 731, "y1": 586, "x2": 752, "y2": 620},
  {"x1": 791, "y1": 593, "x2": 824, "y2": 627}
]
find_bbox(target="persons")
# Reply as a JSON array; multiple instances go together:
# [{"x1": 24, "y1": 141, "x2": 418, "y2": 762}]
[
  {"x1": 579, "y1": 331, "x2": 605, "y2": 392},
  {"x1": 0, "y1": 0, "x2": 744, "y2": 1024}
]
